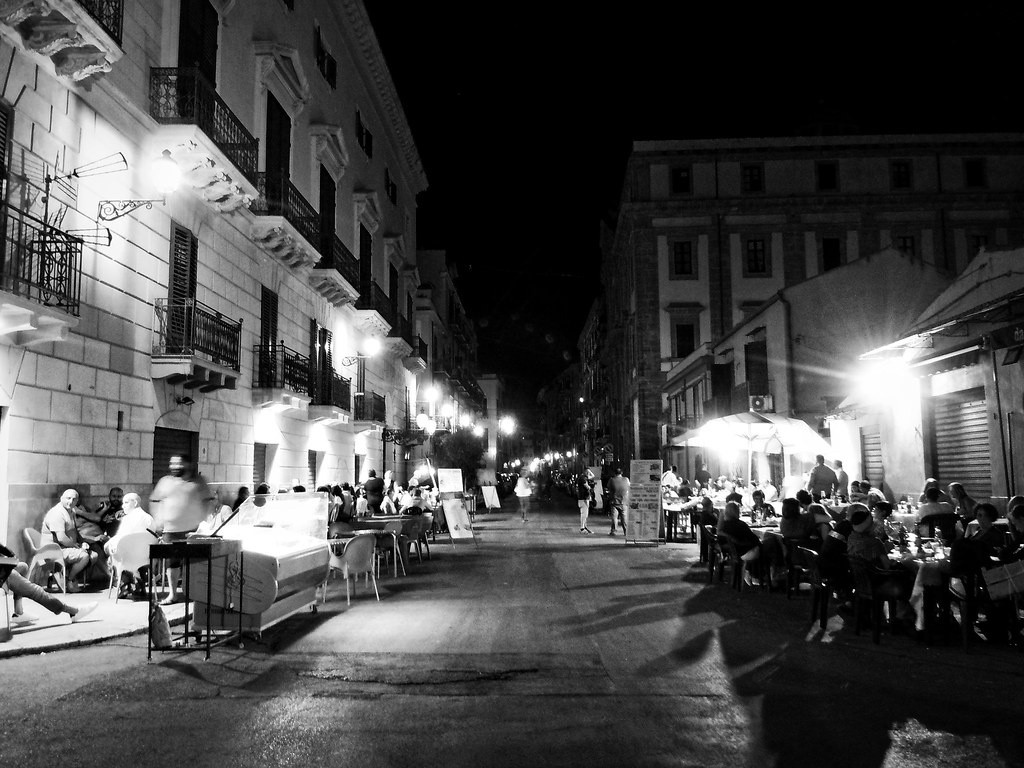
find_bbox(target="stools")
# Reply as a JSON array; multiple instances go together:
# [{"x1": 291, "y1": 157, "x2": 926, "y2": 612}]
[{"x1": 0, "y1": 579, "x2": 13, "y2": 640}]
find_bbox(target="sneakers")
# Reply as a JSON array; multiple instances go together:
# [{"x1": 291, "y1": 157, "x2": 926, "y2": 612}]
[
  {"x1": 67, "y1": 578, "x2": 81, "y2": 593},
  {"x1": 578, "y1": 527, "x2": 594, "y2": 534},
  {"x1": 53, "y1": 572, "x2": 66, "y2": 592},
  {"x1": 743, "y1": 571, "x2": 758, "y2": 585},
  {"x1": 68, "y1": 599, "x2": 96, "y2": 623},
  {"x1": 118, "y1": 584, "x2": 146, "y2": 600},
  {"x1": 11, "y1": 612, "x2": 37, "y2": 627}
]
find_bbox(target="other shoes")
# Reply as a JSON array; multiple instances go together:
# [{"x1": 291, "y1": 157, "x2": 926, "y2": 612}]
[
  {"x1": 607, "y1": 532, "x2": 617, "y2": 536},
  {"x1": 520, "y1": 518, "x2": 528, "y2": 523},
  {"x1": 160, "y1": 597, "x2": 180, "y2": 605},
  {"x1": 621, "y1": 533, "x2": 627, "y2": 536}
]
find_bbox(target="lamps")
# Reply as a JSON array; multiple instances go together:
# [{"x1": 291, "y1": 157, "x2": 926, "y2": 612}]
[
  {"x1": 176, "y1": 396, "x2": 195, "y2": 406},
  {"x1": 98, "y1": 147, "x2": 177, "y2": 221}
]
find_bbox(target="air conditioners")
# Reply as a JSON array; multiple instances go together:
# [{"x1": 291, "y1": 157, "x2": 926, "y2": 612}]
[{"x1": 749, "y1": 395, "x2": 774, "y2": 412}]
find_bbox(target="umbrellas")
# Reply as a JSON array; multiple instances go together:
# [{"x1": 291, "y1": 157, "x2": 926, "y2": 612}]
[{"x1": 671, "y1": 411, "x2": 836, "y2": 503}]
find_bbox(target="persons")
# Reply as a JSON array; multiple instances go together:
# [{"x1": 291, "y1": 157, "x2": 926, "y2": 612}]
[
  {"x1": 833, "y1": 460, "x2": 849, "y2": 502},
  {"x1": 948, "y1": 482, "x2": 982, "y2": 531},
  {"x1": 148, "y1": 452, "x2": 215, "y2": 605},
  {"x1": 102, "y1": 493, "x2": 157, "y2": 598},
  {"x1": 71, "y1": 504, "x2": 125, "y2": 588},
  {"x1": 661, "y1": 462, "x2": 1023, "y2": 648},
  {"x1": 364, "y1": 469, "x2": 385, "y2": 515},
  {"x1": 208, "y1": 469, "x2": 447, "y2": 555},
  {"x1": 576, "y1": 467, "x2": 596, "y2": 534},
  {"x1": 94, "y1": 487, "x2": 124, "y2": 538},
  {"x1": 805, "y1": 453, "x2": 840, "y2": 504},
  {"x1": 917, "y1": 488, "x2": 955, "y2": 537},
  {"x1": 694, "y1": 464, "x2": 712, "y2": 489},
  {"x1": 513, "y1": 470, "x2": 533, "y2": 522},
  {"x1": 231, "y1": 486, "x2": 250, "y2": 511},
  {"x1": 719, "y1": 501, "x2": 783, "y2": 586},
  {"x1": 0, "y1": 543, "x2": 99, "y2": 622},
  {"x1": 39, "y1": 488, "x2": 98, "y2": 592},
  {"x1": 606, "y1": 468, "x2": 630, "y2": 535}
]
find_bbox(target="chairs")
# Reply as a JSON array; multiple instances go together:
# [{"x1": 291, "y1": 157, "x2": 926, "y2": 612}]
[
  {"x1": 323, "y1": 494, "x2": 475, "y2": 607},
  {"x1": 24, "y1": 527, "x2": 86, "y2": 594},
  {"x1": 658, "y1": 482, "x2": 1023, "y2": 649},
  {"x1": 108, "y1": 530, "x2": 159, "y2": 604}
]
